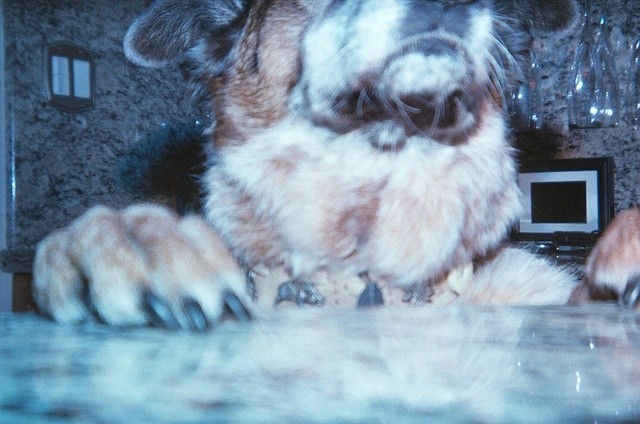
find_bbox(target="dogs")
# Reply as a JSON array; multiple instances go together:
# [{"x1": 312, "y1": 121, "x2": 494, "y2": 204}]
[{"x1": 30, "y1": 0, "x2": 640, "y2": 336}]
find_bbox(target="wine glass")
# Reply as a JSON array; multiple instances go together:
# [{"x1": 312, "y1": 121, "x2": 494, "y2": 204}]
[
  {"x1": 599, "y1": 0, "x2": 621, "y2": 124},
  {"x1": 625, "y1": 35, "x2": 640, "y2": 131},
  {"x1": 503, "y1": 1, "x2": 542, "y2": 130},
  {"x1": 568, "y1": 0, "x2": 603, "y2": 130}
]
